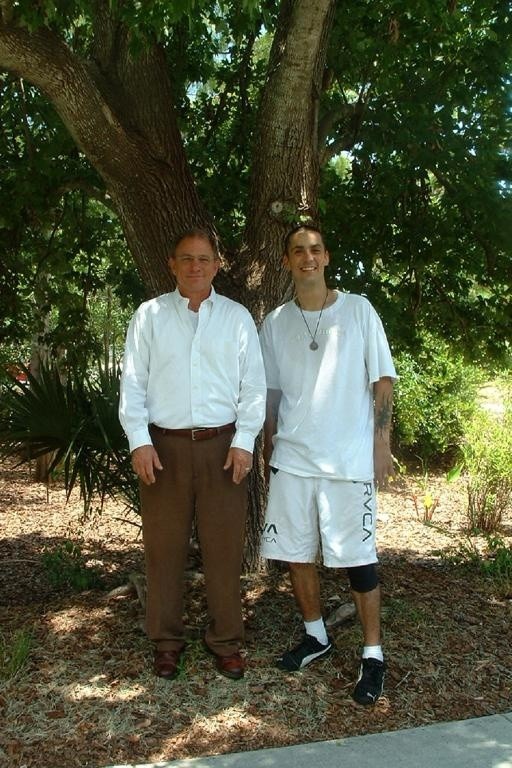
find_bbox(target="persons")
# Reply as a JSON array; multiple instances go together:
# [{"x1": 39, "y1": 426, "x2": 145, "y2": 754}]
[
  {"x1": 116, "y1": 227, "x2": 270, "y2": 679},
  {"x1": 256, "y1": 227, "x2": 402, "y2": 711}
]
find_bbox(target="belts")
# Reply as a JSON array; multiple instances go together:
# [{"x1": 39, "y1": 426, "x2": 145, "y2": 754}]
[{"x1": 148, "y1": 421, "x2": 236, "y2": 441}]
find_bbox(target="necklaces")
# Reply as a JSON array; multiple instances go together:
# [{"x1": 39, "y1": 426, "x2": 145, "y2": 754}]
[{"x1": 294, "y1": 288, "x2": 331, "y2": 351}]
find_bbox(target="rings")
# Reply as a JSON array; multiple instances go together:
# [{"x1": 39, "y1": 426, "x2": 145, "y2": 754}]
[{"x1": 245, "y1": 467, "x2": 250, "y2": 471}]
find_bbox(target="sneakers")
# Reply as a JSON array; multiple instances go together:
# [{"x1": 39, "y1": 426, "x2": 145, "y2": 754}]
[
  {"x1": 275, "y1": 629, "x2": 333, "y2": 672},
  {"x1": 351, "y1": 654, "x2": 386, "y2": 705}
]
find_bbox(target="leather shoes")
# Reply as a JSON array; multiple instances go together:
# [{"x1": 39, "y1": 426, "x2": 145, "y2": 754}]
[
  {"x1": 202, "y1": 639, "x2": 244, "y2": 680},
  {"x1": 154, "y1": 650, "x2": 180, "y2": 677}
]
